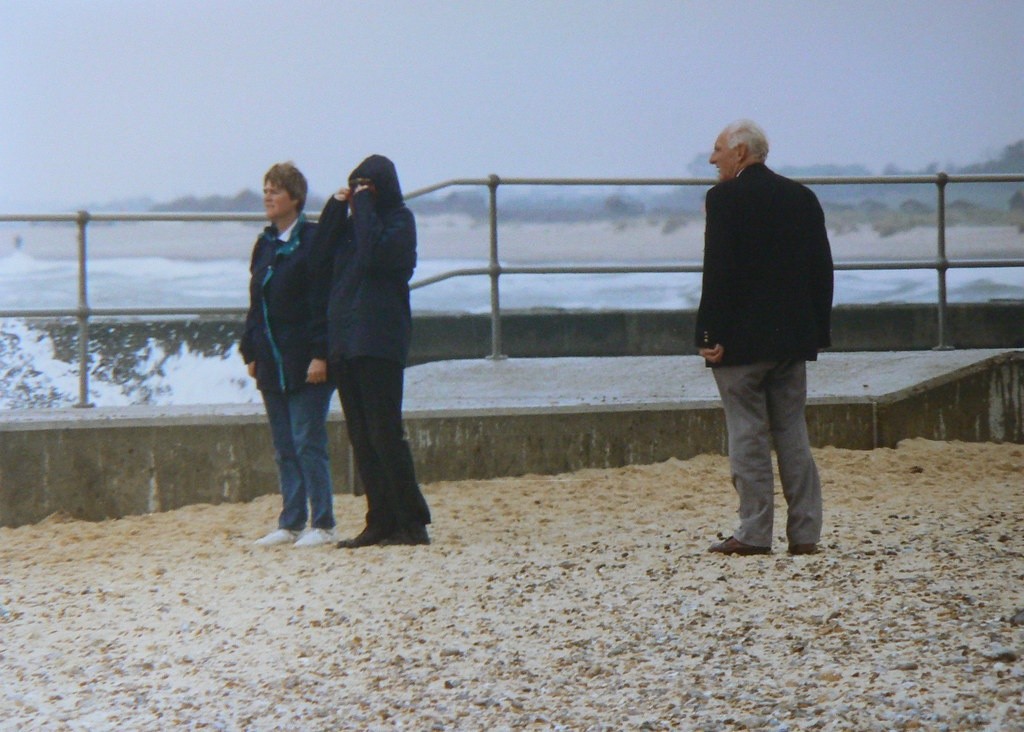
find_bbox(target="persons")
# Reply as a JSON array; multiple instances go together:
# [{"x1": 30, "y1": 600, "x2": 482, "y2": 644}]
[
  {"x1": 309, "y1": 154, "x2": 432, "y2": 548},
  {"x1": 693, "y1": 118, "x2": 836, "y2": 556},
  {"x1": 238, "y1": 164, "x2": 337, "y2": 550}
]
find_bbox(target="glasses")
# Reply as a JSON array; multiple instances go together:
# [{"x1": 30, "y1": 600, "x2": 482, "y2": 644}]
[{"x1": 347, "y1": 179, "x2": 371, "y2": 188}]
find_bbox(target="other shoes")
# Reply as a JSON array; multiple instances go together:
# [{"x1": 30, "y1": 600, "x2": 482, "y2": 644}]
[
  {"x1": 292, "y1": 527, "x2": 337, "y2": 546},
  {"x1": 337, "y1": 523, "x2": 431, "y2": 548},
  {"x1": 788, "y1": 541, "x2": 818, "y2": 555},
  {"x1": 254, "y1": 529, "x2": 305, "y2": 546},
  {"x1": 707, "y1": 535, "x2": 771, "y2": 555}
]
